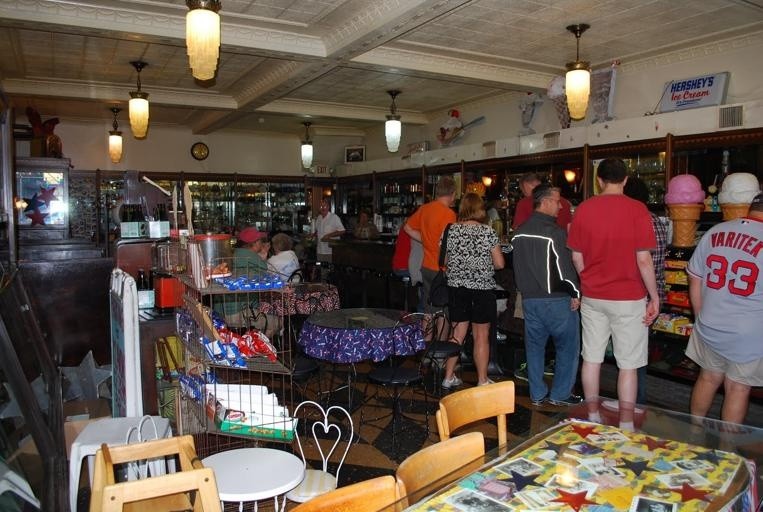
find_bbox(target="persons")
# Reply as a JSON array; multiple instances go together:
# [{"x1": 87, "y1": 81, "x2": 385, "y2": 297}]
[
  {"x1": 255, "y1": 231, "x2": 273, "y2": 262},
  {"x1": 685, "y1": 192, "x2": 763, "y2": 455},
  {"x1": 266, "y1": 232, "x2": 303, "y2": 284},
  {"x1": 211, "y1": 226, "x2": 285, "y2": 339},
  {"x1": 305, "y1": 199, "x2": 346, "y2": 281},
  {"x1": 394, "y1": 177, "x2": 504, "y2": 388},
  {"x1": 514, "y1": 157, "x2": 668, "y2": 433},
  {"x1": 351, "y1": 210, "x2": 381, "y2": 240}
]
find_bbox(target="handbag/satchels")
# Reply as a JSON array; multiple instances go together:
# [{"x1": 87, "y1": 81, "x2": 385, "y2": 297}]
[{"x1": 439, "y1": 223, "x2": 452, "y2": 267}]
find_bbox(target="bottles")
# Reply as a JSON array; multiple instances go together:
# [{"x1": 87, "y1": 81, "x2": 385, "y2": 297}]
[{"x1": 383, "y1": 182, "x2": 424, "y2": 205}]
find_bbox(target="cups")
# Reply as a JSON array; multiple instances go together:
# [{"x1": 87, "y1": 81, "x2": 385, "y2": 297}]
[{"x1": 622, "y1": 156, "x2": 664, "y2": 174}]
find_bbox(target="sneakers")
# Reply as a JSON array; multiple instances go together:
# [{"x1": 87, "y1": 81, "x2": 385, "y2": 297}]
[
  {"x1": 542, "y1": 364, "x2": 555, "y2": 375},
  {"x1": 532, "y1": 397, "x2": 547, "y2": 406},
  {"x1": 514, "y1": 363, "x2": 529, "y2": 382},
  {"x1": 603, "y1": 400, "x2": 647, "y2": 414},
  {"x1": 477, "y1": 378, "x2": 498, "y2": 387},
  {"x1": 548, "y1": 395, "x2": 584, "y2": 406},
  {"x1": 441, "y1": 376, "x2": 465, "y2": 390}
]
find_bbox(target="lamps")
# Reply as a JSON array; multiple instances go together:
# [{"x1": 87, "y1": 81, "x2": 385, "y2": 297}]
[
  {"x1": 191, "y1": 142, "x2": 209, "y2": 160},
  {"x1": 108, "y1": 107, "x2": 123, "y2": 163},
  {"x1": 385, "y1": 89, "x2": 403, "y2": 152},
  {"x1": 128, "y1": 60, "x2": 151, "y2": 139},
  {"x1": 564, "y1": 23, "x2": 592, "y2": 120},
  {"x1": 301, "y1": 121, "x2": 314, "y2": 170},
  {"x1": 184, "y1": 0, "x2": 221, "y2": 80}
]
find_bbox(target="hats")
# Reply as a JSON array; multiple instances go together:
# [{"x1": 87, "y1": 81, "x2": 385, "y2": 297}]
[{"x1": 240, "y1": 227, "x2": 269, "y2": 243}]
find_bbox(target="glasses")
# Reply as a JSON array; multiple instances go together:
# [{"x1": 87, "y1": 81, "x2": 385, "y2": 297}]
[{"x1": 320, "y1": 205, "x2": 327, "y2": 209}]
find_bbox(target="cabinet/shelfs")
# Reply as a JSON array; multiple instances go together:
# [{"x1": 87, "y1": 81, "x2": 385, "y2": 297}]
[{"x1": 96, "y1": 169, "x2": 331, "y2": 260}]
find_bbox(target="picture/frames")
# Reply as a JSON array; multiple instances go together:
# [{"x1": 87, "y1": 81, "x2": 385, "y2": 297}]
[{"x1": 658, "y1": 70, "x2": 730, "y2": 113}]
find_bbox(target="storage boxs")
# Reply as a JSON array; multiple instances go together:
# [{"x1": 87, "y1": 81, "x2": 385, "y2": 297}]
[
  {"x1": 63, "y1": 416, "x2": 110, "y2": 489},
  {"x1": 5, "y1": 439, "x2": 46, "y2": 488}
]
